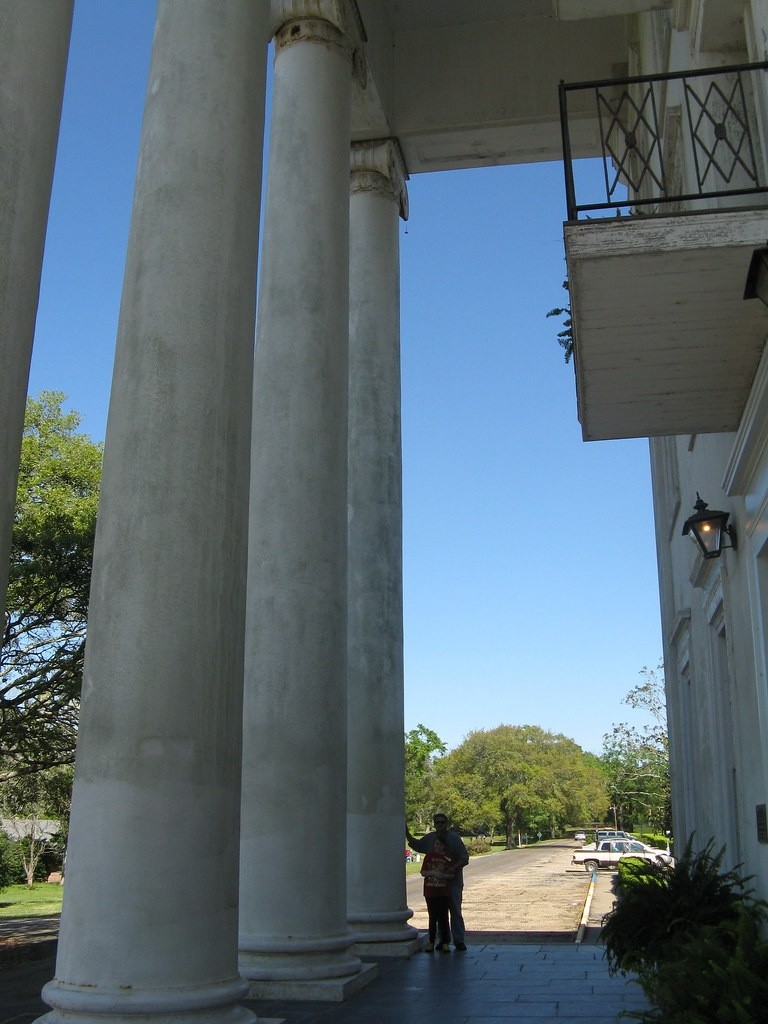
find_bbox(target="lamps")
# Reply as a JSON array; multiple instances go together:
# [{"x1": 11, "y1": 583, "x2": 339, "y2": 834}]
[{"x1": 681, "y1": 491, "x2": 738, "y2": 559}]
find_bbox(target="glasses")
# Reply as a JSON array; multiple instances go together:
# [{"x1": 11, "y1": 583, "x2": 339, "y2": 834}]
[{"x1": 434, "y1": 820, "x2": 447, "y2": 825}]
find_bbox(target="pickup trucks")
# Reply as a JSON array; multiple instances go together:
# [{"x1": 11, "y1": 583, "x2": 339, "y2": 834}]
[{"x1": 571, "y1": 840, "x2": 673, "y2": 872}]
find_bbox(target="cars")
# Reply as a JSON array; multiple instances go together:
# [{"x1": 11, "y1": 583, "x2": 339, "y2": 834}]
[
  {"x1": 581, "y1": 838, "x2": 640, "y2": 849},
  {"x1": 574, "y1": 831, "x2": 586, "y2": 840}
]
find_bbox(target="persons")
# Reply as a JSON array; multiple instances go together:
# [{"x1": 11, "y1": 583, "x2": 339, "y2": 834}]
[{"x1": 406, "y1": 814, "x2": 469, "y2": 952}]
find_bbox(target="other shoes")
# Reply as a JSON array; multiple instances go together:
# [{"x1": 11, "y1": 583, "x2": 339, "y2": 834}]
[
  {"x1": 456, "y1": 943, "x2": 467, "y2": 951},
  {"x1": 425, "y1": 943, "x2": 434, "y2": 953},
  {"x1": 443, "y1": 944, "x2": 450, "y2": 952},
  {"x1": 435, "y1": 943, "x2": 443, "y2": 951}
]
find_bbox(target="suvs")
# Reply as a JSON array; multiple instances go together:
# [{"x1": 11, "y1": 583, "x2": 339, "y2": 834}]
[{"x1": 592, "y1": 830, "x2": 637, "y2": 844}]
